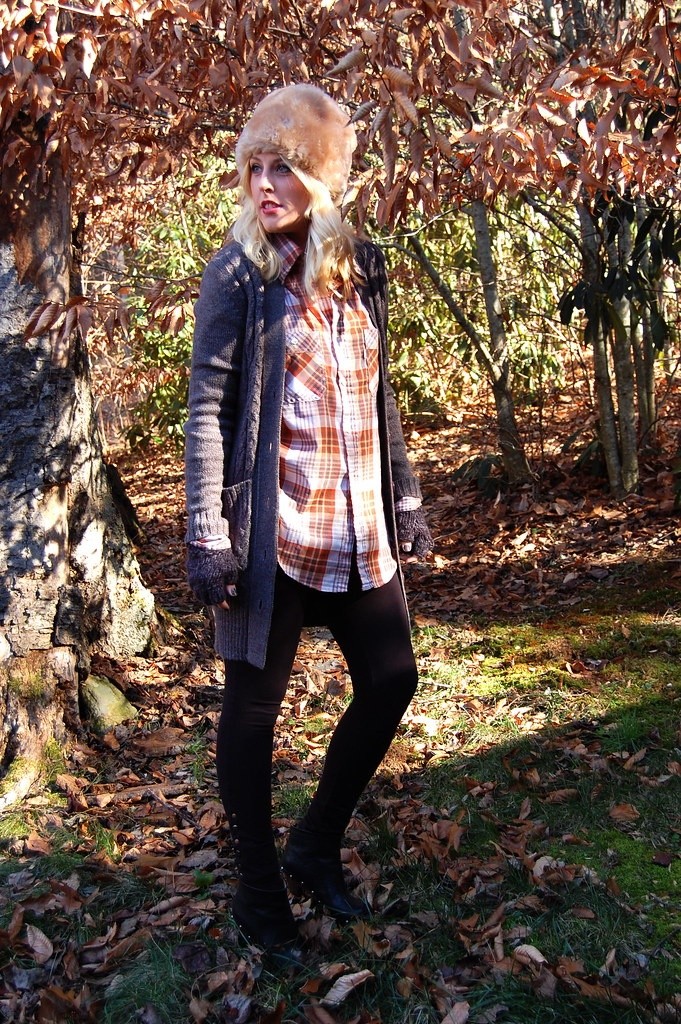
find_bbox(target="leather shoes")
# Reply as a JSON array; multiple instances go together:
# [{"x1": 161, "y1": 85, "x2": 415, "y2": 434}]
[
  {"x1": 232, "y1": 881, "x2": 323, "y2": 978},
  {"x1": 282, "y1": 825, "x2": 374, "y2": 925}
]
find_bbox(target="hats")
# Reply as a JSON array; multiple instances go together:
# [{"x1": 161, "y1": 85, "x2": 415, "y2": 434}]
[{"x1": 234, "y1": 84, "x2": 357, "y2": 207}]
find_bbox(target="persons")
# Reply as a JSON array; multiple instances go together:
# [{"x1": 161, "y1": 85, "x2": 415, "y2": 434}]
[{"x1": 182, "y1": 82, "x2": 439, "y2": 975}]
